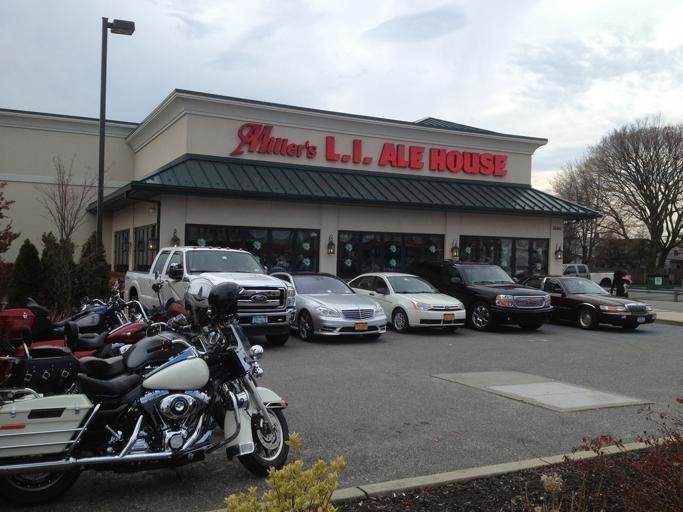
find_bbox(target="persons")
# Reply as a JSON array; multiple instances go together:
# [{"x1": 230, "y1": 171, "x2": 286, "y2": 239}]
[
  {"x1": 621, "y1": 269, "x2": 632, "y2": 294},
  {"x1": 609, "y1": 271, "x2": 627, "y2": 299}
]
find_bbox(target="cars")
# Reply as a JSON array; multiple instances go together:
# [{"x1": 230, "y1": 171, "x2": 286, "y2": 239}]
[
  {"x1": 345, "y1": 271, "x2": 466, "y2": 333},
  {"x1": 404, "y1": 260, "x2": 554, "y2": 332},
  {"x1": 267, "y1": 270, "x2": 387, "y2": 342},
  {"x1": 517, "y1": 275, "x2": 656, "y2": 329}
]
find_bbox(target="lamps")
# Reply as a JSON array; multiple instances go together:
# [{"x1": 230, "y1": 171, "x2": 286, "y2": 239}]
[
  {"x1": 555, "y1": 243, "x2": 564, "y2": 259},
  {"x1": 452, "y1": 239, "x2": 459, "y2": 258},
  {"x1": 327, "y1": 234, "x2": 336, "y2": 256}
]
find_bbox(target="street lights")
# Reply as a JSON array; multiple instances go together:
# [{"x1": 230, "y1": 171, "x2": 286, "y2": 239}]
[{"x1": 96, "y1": 16, "x2": 135, "y2": 241}]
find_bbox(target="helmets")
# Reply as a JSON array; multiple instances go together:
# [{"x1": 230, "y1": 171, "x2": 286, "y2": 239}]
[
  {"x1": 184, "y1": 277, "x2": 215, "y2": 309},
  {"x1": 207, "y1": 281, "x2": 255, "y2": 313}
]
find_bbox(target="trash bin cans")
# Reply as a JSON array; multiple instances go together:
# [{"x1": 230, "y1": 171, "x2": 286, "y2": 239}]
[{"x1": 647, "y1": 275, "x2": 672, "y2": 289}]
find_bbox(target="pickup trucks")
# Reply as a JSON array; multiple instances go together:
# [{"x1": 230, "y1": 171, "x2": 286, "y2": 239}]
[
  {"x1": 122, "y1": 245, "x2": 296, "y2": 345},
  {"x1": 561, "y1": 262, "x2": 614, "y2": 287}
]
